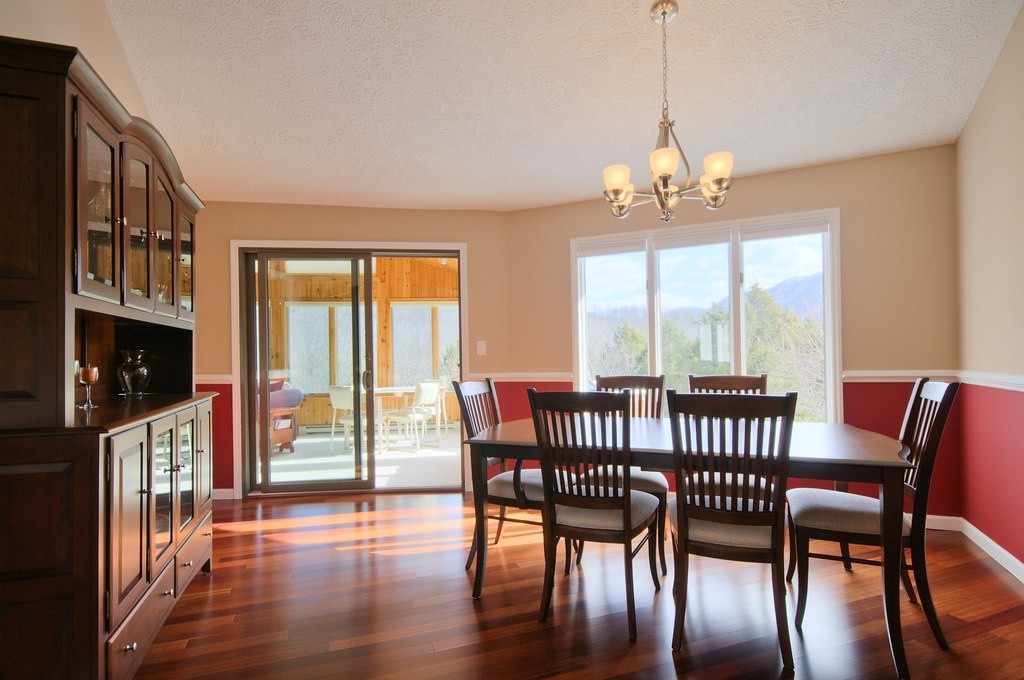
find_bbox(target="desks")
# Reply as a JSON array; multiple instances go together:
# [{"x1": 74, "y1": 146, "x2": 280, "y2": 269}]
[
  {"x1": 462, "y1": 415, "x2": 917, "y2": 680},
  {"x1": 361, "y1": 384, "x2": 416, "y2": 446}
]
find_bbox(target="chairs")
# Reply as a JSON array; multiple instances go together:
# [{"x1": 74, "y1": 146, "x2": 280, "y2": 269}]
[
  {"x1": 786, "y1": 377, "x2": 963, "y2": 650},
  {"x1": 577, "y1": 373, "x2": 667, "y2": 574},
  {"x1": 451, "y1": 378, "x2": 578, "y2": 575},
  {"x1": 329, "y1": 376, "x2": 448, "y2": 453},
  {"x1": 666, "y1": 389, "x2": 797, "y2": 668},
  {"x1": 256, "y1": 381, "x2": 304, "y2": 457},
  {"x1": 685, "y1": 374, "x2": 774, "y2": 500},
  {"x1": 528, "y1": 387, "x2": 660, "y2": 642}
]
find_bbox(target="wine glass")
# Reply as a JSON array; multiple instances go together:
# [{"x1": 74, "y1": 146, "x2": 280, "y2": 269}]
[{"x1": 78, "y1": 367, "x2": 98, "y2": 409}]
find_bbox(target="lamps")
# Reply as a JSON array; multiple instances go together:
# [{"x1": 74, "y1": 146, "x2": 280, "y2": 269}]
[{"x1": 602, "y1": 1, "x2": 735, "y2": 224}]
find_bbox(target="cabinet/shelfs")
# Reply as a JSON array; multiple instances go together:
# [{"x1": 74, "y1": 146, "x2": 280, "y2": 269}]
[{"x1": 0, "y1": 35, "x2": 221, "y2": 680}]
